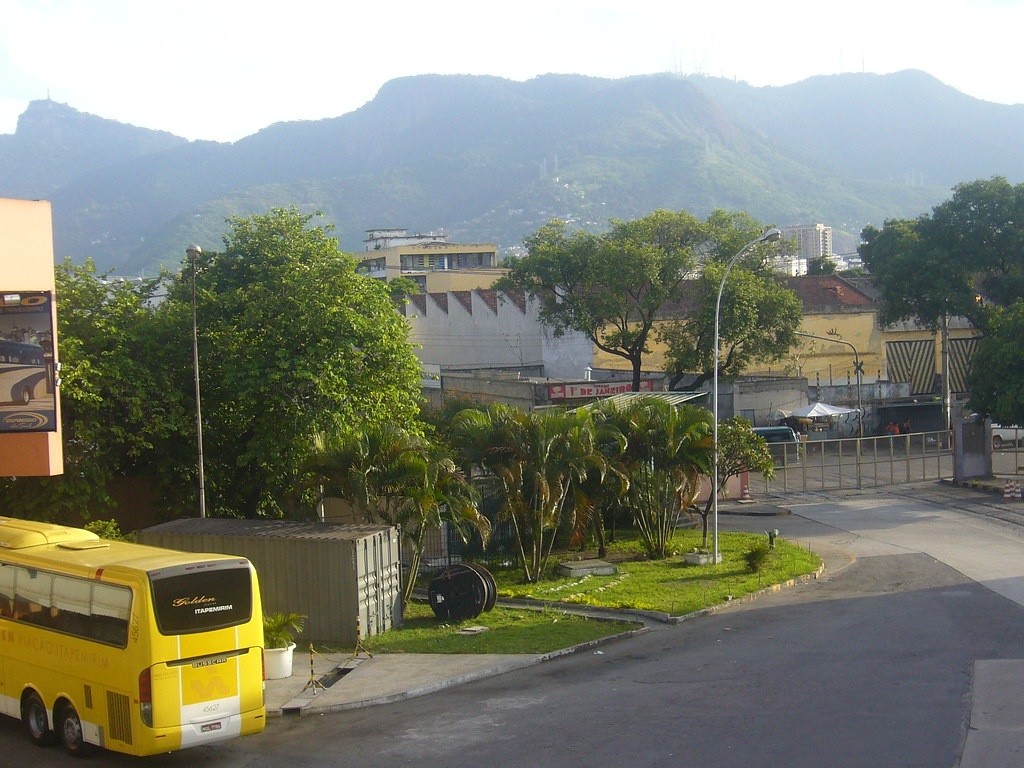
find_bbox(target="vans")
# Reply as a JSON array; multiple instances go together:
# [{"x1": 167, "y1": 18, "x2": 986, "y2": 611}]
[{"x1": 752, "y1": 428, "x2": 801, "y2": 470}]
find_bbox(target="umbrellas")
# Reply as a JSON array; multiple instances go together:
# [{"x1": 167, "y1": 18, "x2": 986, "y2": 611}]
[{"x1": 766, "y1": 409, "x2": 792, "y2": 421}]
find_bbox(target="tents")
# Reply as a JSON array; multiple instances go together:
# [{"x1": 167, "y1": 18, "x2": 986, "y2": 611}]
[{"x1": 792, "y1": 402, "x2": 862, "y2": 437}]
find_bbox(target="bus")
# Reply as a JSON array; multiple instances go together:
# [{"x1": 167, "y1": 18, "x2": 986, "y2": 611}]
[{"x1": 0, "y1": 513, "x2": 268, "y2": 759}]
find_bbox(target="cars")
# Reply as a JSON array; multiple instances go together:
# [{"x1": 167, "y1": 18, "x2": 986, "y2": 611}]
[{"x1": 964, "y1": 412, "x2": 1024, "y2": 449}]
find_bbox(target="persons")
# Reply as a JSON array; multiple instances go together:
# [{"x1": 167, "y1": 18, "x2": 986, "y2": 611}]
[{"x1": 885, "y1": 417, "x2": 911, "y2": 448}]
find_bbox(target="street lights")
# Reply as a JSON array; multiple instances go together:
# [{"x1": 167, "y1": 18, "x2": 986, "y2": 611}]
[
  {"x1": 792, "y1": 330, "x2": 863, "y2": 455},
  {"x1": 711, "y1": 227, "x2": 781, "y2": 565},
  {"x1": 184, "y1": 241, "x2": 208, "y2": 522}
]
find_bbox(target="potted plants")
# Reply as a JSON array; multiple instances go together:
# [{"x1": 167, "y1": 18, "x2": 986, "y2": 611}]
[{"x1": 262, "y1": 609, "x2": 309, "y2": 680}]
